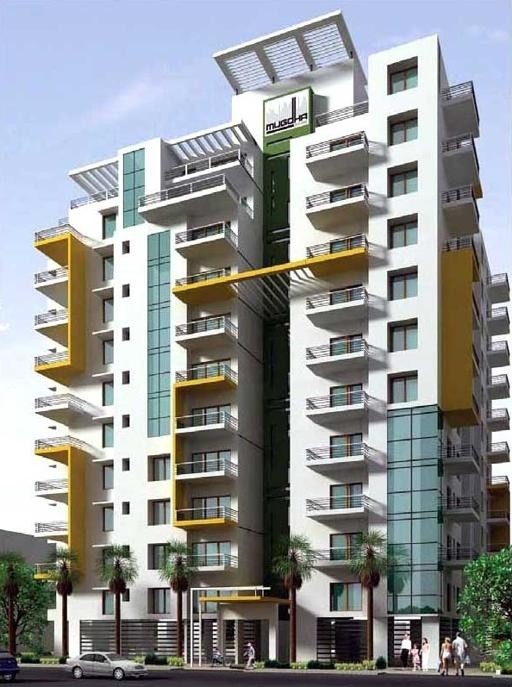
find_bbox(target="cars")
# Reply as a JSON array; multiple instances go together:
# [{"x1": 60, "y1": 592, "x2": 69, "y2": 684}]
[
  {"x1": 64, "y1": 649, "x2": 149, "y2": 680},
  {"x1": 0, "y1": 649, "x2": 21, "y2": 681}
]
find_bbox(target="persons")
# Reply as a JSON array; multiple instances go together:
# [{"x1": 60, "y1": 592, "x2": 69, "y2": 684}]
[
  {"x1": 243, "y1": 642, "x2": 256, "y2": 669},
  {"x1": 399, "y1": 632, "x2": 412, "y2": 670},
  {"x1": 418, "y1": 636, "x2": 430, "y2": 673},
  {"x1": 450, "y1": 631, "x2": 468, "y2": 677},
  {"x1": 439, "y1": 635, "x2": 453, "y2": 676},
  {"x1": 210, "y1": 646, "x2": 223, "y2": 667},
  {"x1": 410, "y1": 642, "x2": 421, "y2": 671}
]
